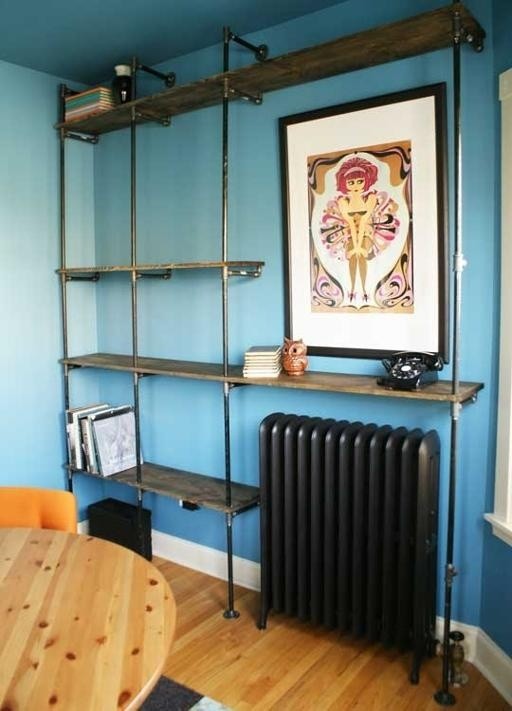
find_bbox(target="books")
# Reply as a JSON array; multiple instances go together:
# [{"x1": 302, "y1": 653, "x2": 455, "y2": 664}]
[
  {"x1": 66, "y1": 403, "x2": 143, "y2": 478},
  {"x1": 243, "y1": 346, "x2": 283, "y2": 378},
  {"x1": 65, "y1": 87, "x2": 115, "y2": 123}
]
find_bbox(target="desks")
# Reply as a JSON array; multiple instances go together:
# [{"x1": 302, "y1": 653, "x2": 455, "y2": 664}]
[{"x1": 0, "y1": 525, "x2": 182, "y2": 711}]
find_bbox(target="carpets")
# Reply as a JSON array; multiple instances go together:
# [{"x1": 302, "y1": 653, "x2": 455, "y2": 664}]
[{"x1": 132, "y1": 670, "x2": 236, "y2": 711}]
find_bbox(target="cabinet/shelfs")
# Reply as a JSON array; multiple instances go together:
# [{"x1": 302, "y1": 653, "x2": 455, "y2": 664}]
[{"x1": 54, "y1": 1, "x2": 489, "y2": 711}]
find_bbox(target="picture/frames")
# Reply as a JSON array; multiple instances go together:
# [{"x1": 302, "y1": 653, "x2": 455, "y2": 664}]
[{"x1": 274, "y1": 79, "x2": 452, "y2": 368}]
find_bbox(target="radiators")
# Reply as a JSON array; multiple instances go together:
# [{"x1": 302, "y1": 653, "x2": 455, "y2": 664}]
[{"x1": 252, "y1": 406, "x2": 449, "y2": 694}]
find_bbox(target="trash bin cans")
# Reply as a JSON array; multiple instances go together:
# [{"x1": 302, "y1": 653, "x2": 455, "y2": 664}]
[{"x1": 88, "y1": 497, "x2": 152, "y2": 560}]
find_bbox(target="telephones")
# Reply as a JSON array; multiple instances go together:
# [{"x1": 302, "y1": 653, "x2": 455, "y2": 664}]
[{"x1": 376, "y1": 352, "x2": 444, "y2": 390}]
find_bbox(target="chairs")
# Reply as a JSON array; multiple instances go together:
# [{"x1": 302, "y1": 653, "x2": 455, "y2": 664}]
[{"x1": 0, "y1": 485, "x2": 80, "y2": 536}]
